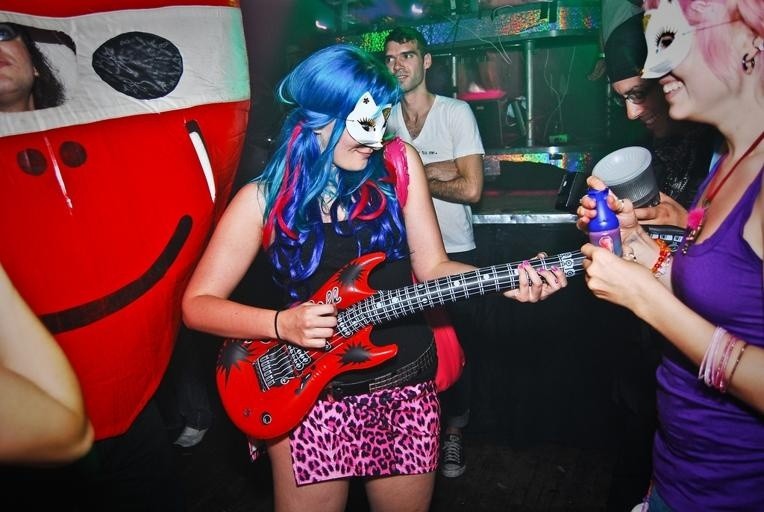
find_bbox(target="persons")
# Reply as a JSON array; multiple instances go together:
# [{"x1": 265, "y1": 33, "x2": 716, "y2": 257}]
[
  {"x1": 581, "y1": 0, "x2": 764, "y2": 510},
  {"x1": 0, "y1": 21, "x2": 65, "y2": 112},
  {"x1": 180, "y1": 43, "x2": 567, "y2": 512},
  {"x1": 0, "y1": 262, "x2": 96, "y2": 468},
  {"x1": 605, "y1": 10, "x2": 717, "y2": 232},
  {"x1": 379, "y1": 26, "x2": 487, "y2": 478}
]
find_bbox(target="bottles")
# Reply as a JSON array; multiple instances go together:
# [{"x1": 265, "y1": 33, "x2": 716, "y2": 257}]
[{"x1": 579, "y1": 184, "x2": 623, "y2": 261}]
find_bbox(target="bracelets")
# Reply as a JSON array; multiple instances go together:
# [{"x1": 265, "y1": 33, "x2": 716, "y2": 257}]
[
  {"x1": 274, "y1": 310, "x2": 286, "y2": 343},
  {"x1": 697, "y1": 325, "x2": 750, "y2": 396},
  {"x1": 648, "y1": 232, "x2": 672, "y2": 284}
]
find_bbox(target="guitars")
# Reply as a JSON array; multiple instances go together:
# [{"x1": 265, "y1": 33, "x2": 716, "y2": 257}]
[{"x1": 215, "y1": 223, "x2": 687, "y2": 440}]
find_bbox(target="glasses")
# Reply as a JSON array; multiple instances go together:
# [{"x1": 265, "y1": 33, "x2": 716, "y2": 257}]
[{"x1": 611, "y1": 88, "x2": 649, "y2": 106}]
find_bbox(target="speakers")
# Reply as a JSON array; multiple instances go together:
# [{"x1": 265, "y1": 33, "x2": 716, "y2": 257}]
[
  {"x1": 468, "y1": 98, "x2": 504, "y2": 150},
  {"x1": 425, "y1": 53, "x2": 457, "y2": 99},
  {"x1": 556, "y1": 172, "x2": 587, "y2": 214}
]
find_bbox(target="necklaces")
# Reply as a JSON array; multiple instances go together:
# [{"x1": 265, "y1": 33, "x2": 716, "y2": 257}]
[{"x1": 681, "y1": 131, "x2": 763, "y2": 258}]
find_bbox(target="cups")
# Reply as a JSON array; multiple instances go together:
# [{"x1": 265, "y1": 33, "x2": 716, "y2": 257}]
[{"x1": 591, "y1": 146, "x2": 660, "y2": 211}]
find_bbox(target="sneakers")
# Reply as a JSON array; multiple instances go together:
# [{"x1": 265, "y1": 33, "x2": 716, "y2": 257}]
[
  {"x1": 172, "y1": 425, "x2": 209, "y2": 448},
  {"x1": 437, "y1": 431, "x2": 467, "y2": 479}
]
